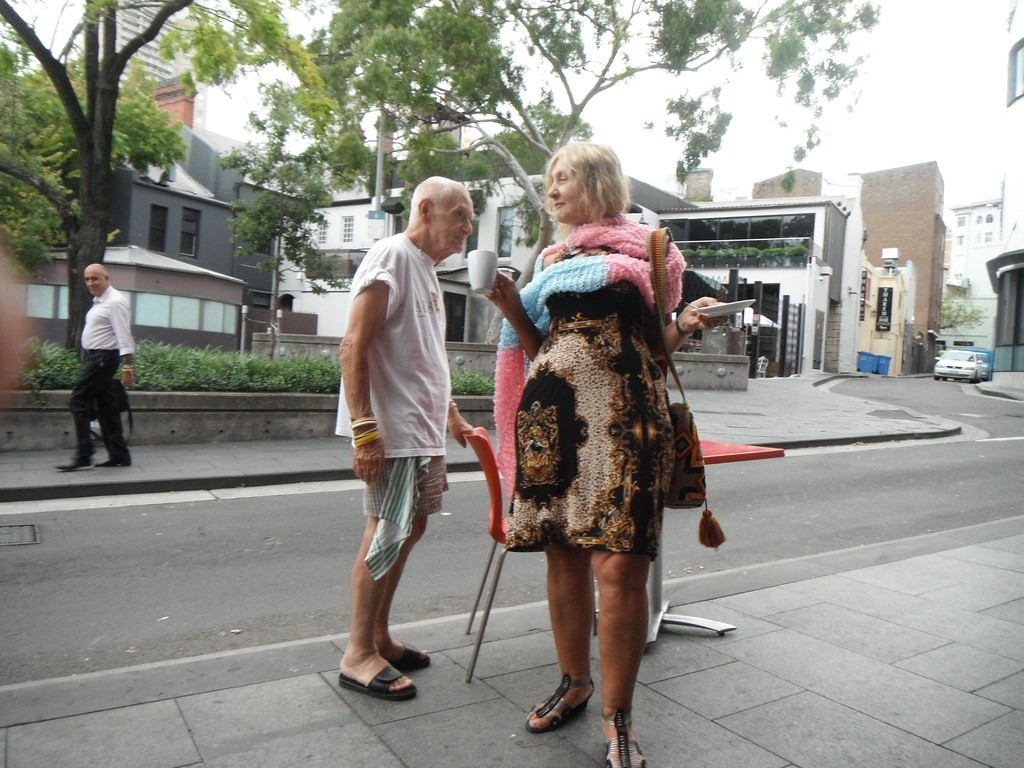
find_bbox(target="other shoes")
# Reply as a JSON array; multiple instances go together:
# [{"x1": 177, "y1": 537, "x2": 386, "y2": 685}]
[
  {"x1": 93, "y1": 450, "x2": 131, "y2": 469},
  {"x1": 56, "y1": 456, "x2": 93, "y2": 471}
]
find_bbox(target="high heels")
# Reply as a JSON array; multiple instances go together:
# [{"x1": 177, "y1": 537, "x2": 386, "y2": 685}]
[
  {"x1": 525, "y1": 674, "x2": 594, "y2": 732},
  {"x1": 601, "y1": 710, "x2": 646, "y2": 768}
]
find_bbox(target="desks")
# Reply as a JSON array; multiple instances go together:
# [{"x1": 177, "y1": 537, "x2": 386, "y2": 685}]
[{"x1": 645, "y1": 441, "x2": 786, "y2": 646}]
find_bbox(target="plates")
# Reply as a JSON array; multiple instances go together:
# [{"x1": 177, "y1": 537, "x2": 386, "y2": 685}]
[{"x1": 691, "y1": 299, "x2": 756, "y2": 318}]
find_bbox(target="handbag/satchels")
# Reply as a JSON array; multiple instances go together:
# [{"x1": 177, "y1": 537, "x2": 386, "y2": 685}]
[
  {"x1": 667, "y1": 403, "x2": 725, "y2": 548},
  {"x1": 87, "y1": 380, "x2": 129, "y2": 421}
]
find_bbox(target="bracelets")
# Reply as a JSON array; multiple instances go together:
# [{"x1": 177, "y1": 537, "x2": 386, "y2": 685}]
[
  {"x1": 675, "y1": 318, "x2": 693, "y2": 338},
  {"x1": 120, "y1": 365, "x2": 134, "y2": 371},
  {"x1": 350, "y1": 416, "x2": 381, "y2": 447}
]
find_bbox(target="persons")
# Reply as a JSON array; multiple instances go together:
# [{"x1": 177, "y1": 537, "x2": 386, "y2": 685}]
[
  {"x1": 483, "y1": 143, "x2": 729, "y2": 768},
  {"x1": 52, "y1": 263, "x2": 136, "y2": 471},
  {"x1": 334, "y1": 177, "x2": 474, "y2": 699}
]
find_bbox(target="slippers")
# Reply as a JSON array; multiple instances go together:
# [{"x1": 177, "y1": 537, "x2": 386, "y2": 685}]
[
  {"x1": 338, "y1": 665, "x2": 417, "y2": 699},
  {"x1": 386, "y1": 641, "x2": 430, "y2": 670}
]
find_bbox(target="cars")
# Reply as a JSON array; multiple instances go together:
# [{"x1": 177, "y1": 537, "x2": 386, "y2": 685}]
[
  {"x1": 974, "y1": 352, "x2": 991, "y2": 381},
  {"x1": 932, "y1": 350, "x2": 984, "y2": 384}
]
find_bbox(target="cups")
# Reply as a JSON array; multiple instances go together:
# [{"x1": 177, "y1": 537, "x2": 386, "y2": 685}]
[{"x1": 467, "y1": 249, "x2": 499, "y2": 294}]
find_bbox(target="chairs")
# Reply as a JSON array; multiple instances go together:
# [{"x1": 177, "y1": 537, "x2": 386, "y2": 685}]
[
  {"x1": 464, "y1": 425, "x2": 598, "y2": 683},
  {"x1": 755, "y1": 356, "x2": 768, "y2": 378}
]
